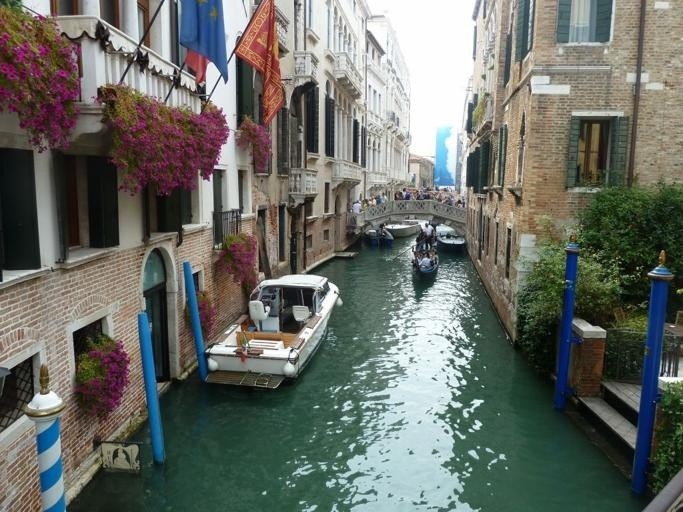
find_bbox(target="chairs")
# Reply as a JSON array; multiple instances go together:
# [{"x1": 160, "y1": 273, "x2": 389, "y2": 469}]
[
  {"x1": 291, "y1": 302, "x2": 311, "y2": 328},
  {"x1": 247, "y1": 299, "x2": 271, "y2": 331}
]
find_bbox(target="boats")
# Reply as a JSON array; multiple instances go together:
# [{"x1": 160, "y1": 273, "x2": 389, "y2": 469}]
[
  {"x1": 362, "y1": 225, "x2": 394, "y2": 250},
  {"x1": 414, "y1": 240, "x2": 438, "y2": 276},
  {"x1": 382, "y1": 219, "x2": 430, "y2": 238},
  {"x1": 434, "y1": 224, "x2": 465, "y2": 250},
  {"x1": 205, "y1": 274, "x2": 343, "y2": 392}
]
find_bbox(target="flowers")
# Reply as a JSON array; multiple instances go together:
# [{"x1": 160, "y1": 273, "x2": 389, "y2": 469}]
[
  {"x1": 234, "y1": 113, "x2": 274, "y2": 171},
  {"x1": 93, "y1": 78, "x2": 231, "y2": 195},
  {"x1": 181, "y1": 289, "x2": 217, "y2": 342},
  {"x1": 73, "y1": 331, "x2": 133, "y2": 425},
  {"x1": 219, "y1": 228, "x2": 261, "y2": 297},
  {"x1": 1, "y1": 0, "x2": 86, "y2": 155}
]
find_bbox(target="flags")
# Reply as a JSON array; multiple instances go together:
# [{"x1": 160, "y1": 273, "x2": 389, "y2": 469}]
[
  {"x1": 179, "y1": 1, "x2": 229, "y2": 83},
  {"x1": 234, "y1": 0, "x2": 285, "y2": 128}
]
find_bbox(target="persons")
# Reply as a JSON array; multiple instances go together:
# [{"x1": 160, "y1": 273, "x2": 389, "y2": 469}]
[
  {"x1": 350, "y1": 186, "x2": 466, "y2": 216},
  {"x1": 416, "y1": 223, "x2": 439, "y2": 268}
]
